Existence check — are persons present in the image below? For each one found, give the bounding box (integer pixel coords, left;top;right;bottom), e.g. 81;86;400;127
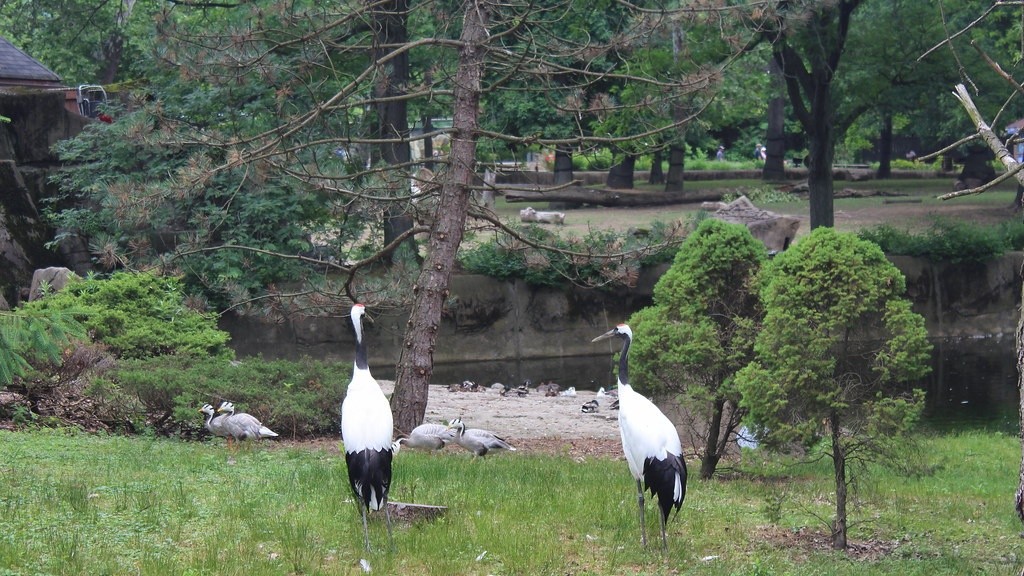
717;145;725;161
754;144;766;160
906;150;916;161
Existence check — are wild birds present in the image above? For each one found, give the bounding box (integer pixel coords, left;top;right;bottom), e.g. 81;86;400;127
341;304;394;570
581;386;613;413
593;324;687;548
563;386;577;397
447;381;511;396
393;419;516;463
514;380;562;398
198;401;279;453
